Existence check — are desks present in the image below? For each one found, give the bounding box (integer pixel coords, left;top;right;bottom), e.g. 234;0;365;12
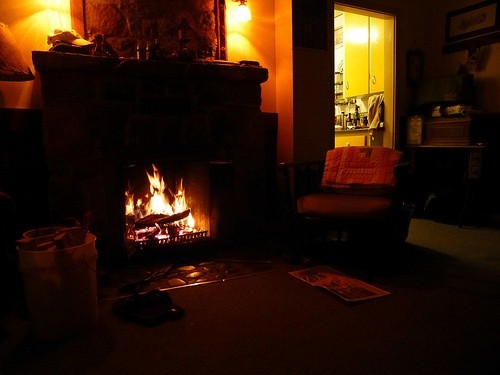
410;144;489;230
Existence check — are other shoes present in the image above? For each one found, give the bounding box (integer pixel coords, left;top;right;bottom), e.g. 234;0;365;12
140;289;183;318
113;296;162;327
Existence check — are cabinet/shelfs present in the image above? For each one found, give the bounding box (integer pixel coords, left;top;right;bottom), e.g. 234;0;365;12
342;11;370;100
334;132;369;148
369;17;385;94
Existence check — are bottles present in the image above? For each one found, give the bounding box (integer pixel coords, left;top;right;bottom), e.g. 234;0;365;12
152;31;161;60
353;105;360;126
346;113;352;130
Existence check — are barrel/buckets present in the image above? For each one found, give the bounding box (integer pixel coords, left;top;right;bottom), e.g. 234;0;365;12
0;80;33;109
18;227;98;341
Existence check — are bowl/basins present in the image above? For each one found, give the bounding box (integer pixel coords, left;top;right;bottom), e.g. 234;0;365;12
348;126;356;130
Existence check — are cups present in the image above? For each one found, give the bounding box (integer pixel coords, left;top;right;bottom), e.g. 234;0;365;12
180;49;197;62
136;39;142;59
145;40;152;61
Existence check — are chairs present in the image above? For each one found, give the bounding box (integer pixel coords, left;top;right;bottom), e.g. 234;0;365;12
279;145;417;271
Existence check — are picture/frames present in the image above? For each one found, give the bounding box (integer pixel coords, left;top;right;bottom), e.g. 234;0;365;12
444;0;500;43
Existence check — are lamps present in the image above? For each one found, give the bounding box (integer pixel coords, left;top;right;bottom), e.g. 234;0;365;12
0;24;35;108
232;0;252;22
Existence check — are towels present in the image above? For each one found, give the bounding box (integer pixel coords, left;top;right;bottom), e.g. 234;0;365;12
368;93;384;140
